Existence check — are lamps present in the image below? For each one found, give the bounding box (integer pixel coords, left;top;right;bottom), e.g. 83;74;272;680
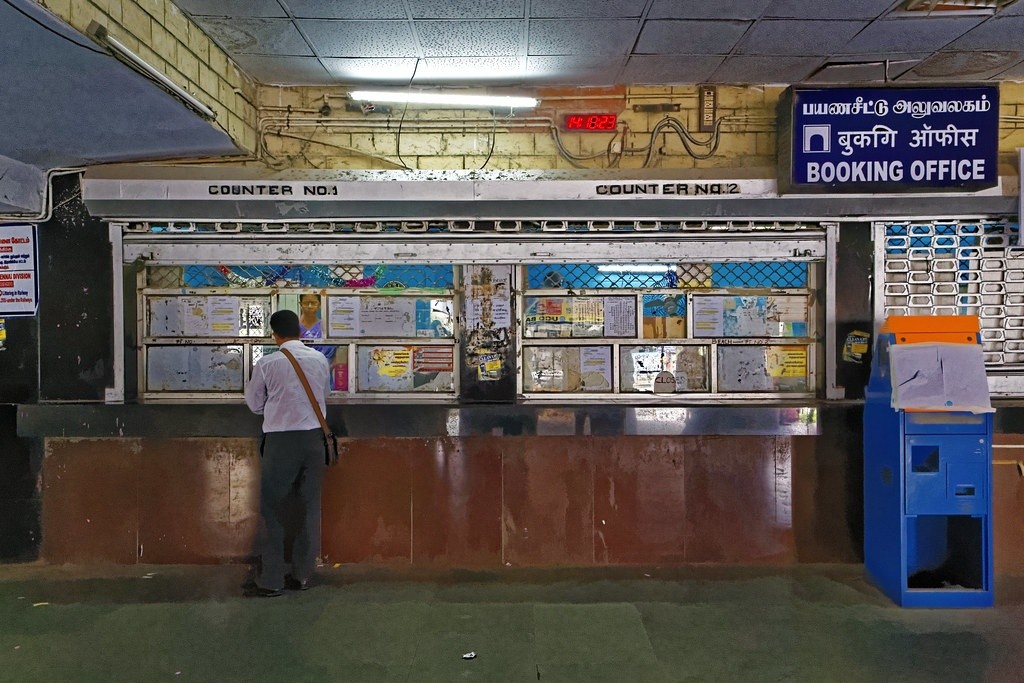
346;89;540;109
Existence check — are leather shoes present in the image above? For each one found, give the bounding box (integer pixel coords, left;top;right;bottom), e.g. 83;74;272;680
245;583;283;596
290;579;310;589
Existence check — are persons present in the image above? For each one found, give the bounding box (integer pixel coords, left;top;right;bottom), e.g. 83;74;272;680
300;294;336;390
244;309;330;596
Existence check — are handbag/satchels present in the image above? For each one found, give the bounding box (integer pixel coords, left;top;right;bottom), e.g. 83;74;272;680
324;432;338;466
